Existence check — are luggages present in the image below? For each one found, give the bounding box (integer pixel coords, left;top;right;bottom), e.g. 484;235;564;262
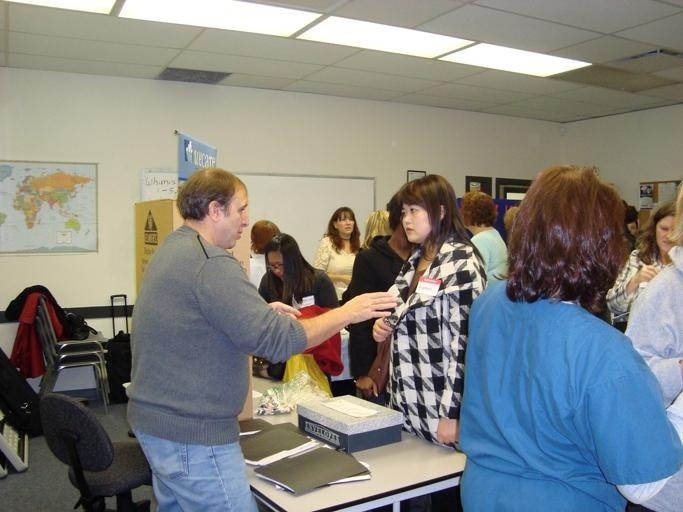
105;294;135;405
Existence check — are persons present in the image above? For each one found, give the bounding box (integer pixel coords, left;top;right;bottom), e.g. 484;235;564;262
624;178;683;444
125;168;398;512
503;206;520;235
363;208;392;250
620;199;637;250
458;163;683;512
314;207;361;300
372;175;489;452
606;202;676;333
342;189;419;406
257;233;339;385
250;219;280;290
459;191;509;287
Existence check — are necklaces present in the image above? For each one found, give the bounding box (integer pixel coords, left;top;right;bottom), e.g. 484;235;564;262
342;239;350;241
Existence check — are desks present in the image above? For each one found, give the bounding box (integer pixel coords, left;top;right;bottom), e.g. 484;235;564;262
123;377;467;512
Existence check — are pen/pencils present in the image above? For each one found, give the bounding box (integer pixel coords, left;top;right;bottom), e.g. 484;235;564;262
639;260;645;266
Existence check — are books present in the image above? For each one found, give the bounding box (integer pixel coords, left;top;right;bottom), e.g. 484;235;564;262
230;415;373;498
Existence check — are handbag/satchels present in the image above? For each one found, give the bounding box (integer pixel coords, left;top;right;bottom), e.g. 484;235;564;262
367;326;393;395
64;309;90;341
252;355;282;379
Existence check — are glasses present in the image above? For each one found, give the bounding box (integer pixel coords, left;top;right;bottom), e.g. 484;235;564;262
265;264;284;270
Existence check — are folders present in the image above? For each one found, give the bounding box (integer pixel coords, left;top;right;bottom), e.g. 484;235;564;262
241;418;372;496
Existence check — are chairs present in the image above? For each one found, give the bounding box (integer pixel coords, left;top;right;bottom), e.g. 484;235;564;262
35;298;109;416
39;394;151;512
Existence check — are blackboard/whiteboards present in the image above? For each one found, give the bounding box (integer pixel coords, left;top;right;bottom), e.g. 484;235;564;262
141;170;376;292
